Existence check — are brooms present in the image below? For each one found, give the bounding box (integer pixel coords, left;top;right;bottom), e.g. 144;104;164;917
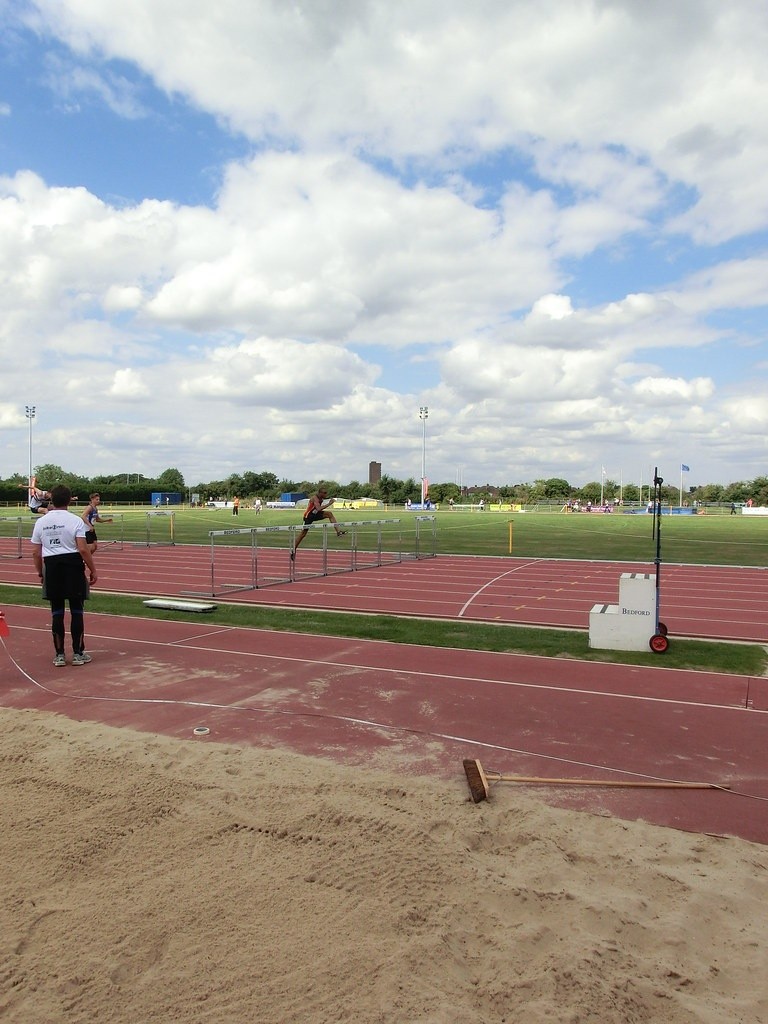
459;759;732;803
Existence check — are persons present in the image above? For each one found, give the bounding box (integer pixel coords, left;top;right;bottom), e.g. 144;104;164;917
254;495;262;515
604;499;610;513
731;503;736;515
191;499;201;507
647;500;653;513
31;485;97;666
233;496;239;516
81;492;113;564
166;496;169;504
479;498;486;510
17;483;78;515
408;497;411;506
613;497;620;506
448;497;455;510
568;498;591;512
499;499;502;511
291;488;348;561
426;497;431;507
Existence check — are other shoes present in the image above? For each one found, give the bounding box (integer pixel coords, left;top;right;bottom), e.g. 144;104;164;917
232;514;238;516
290;549;297;561
337;529;349;538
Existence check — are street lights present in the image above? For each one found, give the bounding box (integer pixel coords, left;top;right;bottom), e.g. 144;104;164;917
25;405;36;506
419;406;428;511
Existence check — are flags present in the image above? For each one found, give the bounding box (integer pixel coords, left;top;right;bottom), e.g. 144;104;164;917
682;464;689;471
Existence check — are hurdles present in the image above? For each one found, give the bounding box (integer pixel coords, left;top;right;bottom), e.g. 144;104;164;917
180;515;439;599
0;510;176;560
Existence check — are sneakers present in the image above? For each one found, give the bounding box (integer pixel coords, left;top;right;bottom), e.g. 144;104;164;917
52;653;66;667
71;651;92;666
